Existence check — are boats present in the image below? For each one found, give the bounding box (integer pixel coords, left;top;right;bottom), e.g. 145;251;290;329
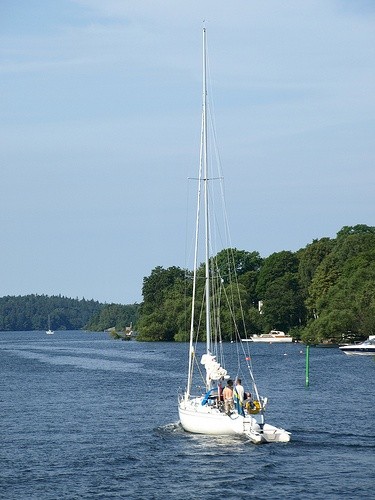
338;334;375;358
239;329;293;344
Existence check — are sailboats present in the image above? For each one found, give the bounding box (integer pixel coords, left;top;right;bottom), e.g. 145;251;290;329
176;17;296;447
45;315;54;335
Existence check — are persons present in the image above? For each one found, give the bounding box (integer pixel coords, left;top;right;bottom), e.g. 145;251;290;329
233;379;245;417
223;380;235;413
